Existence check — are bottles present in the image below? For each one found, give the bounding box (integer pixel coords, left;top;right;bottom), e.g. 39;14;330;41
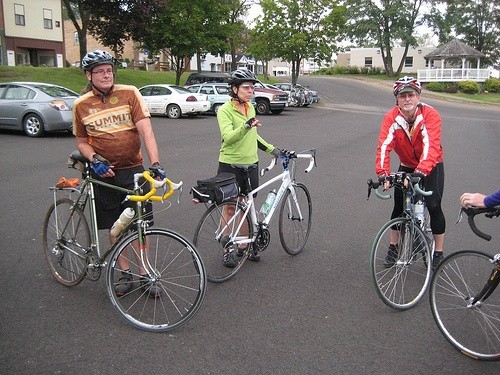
110;207;135;237
415;200;424;227
259;189;277;216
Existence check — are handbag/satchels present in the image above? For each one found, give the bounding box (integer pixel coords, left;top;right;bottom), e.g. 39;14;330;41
193;172;239;204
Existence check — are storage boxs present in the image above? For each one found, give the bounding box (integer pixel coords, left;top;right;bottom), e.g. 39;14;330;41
189;172;241;204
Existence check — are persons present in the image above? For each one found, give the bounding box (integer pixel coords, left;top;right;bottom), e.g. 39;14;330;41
72;49;165;300
459;189;500;209
376;76;445;271
216;67;280;268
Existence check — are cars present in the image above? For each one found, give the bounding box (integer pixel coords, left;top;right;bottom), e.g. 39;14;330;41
181;82;257;117
253;83;320;108
138;83;212;119
0;82;82;137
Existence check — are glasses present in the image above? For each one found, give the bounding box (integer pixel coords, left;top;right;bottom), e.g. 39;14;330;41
237;85;254;91
397;94;417;99
91;70;115;76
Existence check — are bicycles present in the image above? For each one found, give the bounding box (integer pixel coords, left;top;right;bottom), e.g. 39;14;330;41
428;205;500;361
189;148;317;284
41;150;206;332
366;171;435;312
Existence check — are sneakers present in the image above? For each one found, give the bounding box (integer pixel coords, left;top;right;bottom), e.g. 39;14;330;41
383;244;398;267
115;273;133;295
222;250;237;267
141;276;162;298
236;248;260;261
432;253;446;272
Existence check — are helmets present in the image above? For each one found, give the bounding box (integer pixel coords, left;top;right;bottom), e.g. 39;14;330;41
393;76;422;95
82;50;115;71
227;67;258;84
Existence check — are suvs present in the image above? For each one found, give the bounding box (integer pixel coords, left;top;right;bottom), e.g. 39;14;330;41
185;73;289;115
